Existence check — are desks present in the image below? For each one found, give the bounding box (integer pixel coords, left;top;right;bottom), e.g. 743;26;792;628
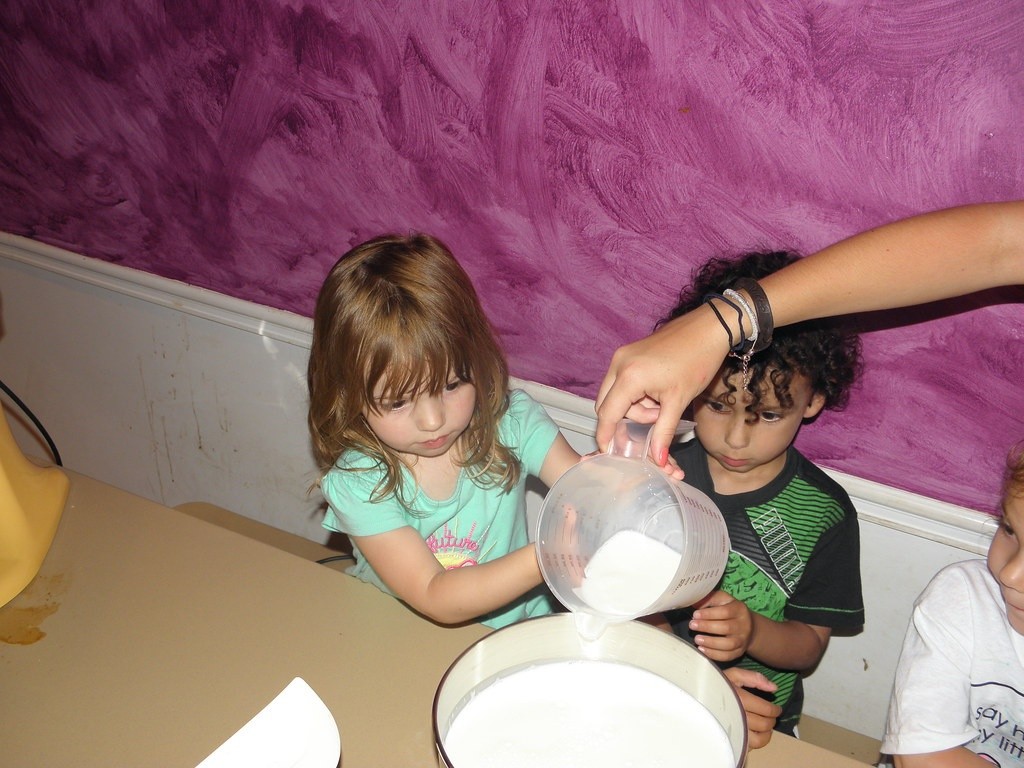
0;452;879;766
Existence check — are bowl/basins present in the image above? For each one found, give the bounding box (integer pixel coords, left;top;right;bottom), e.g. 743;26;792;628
431;611;750;768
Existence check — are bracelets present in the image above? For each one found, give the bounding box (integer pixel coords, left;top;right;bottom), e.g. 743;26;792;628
700;275;776;393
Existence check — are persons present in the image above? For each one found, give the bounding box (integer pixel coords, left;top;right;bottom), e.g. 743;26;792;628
592;199;1024;470
636;244;869;762
876;440;1024;768
304;228;683;635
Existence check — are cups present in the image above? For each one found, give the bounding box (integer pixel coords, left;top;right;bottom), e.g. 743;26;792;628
534;416;731;626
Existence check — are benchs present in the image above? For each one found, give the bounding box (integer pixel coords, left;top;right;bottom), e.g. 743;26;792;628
173;500;884;767
